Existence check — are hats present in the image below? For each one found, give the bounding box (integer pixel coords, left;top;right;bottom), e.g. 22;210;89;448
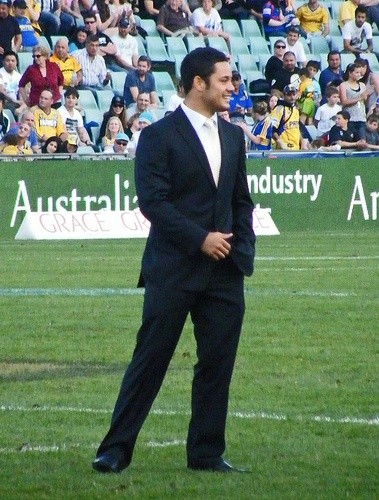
112;96;124;103
283;84;296;95
13;0;28;8
119;18;129;26
232;72;241;79
115;133;129;142
67;135;80;147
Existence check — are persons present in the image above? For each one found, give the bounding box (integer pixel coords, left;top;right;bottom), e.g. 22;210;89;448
0;0;379;156
92;46;256;473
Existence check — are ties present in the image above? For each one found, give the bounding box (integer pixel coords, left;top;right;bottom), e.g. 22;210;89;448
204;119;220;186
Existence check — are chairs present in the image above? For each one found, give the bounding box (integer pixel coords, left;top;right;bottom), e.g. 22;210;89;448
0;0;379;154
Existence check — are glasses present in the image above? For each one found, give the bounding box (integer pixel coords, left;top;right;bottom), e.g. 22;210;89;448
112;104;124;107
85;21;96;24
274;45;286;49
32;54;44;58
231;78;240;81
117;141;127;146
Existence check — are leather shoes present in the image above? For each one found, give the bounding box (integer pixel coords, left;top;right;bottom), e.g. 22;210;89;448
209;460;250;473
91;457;113;473
